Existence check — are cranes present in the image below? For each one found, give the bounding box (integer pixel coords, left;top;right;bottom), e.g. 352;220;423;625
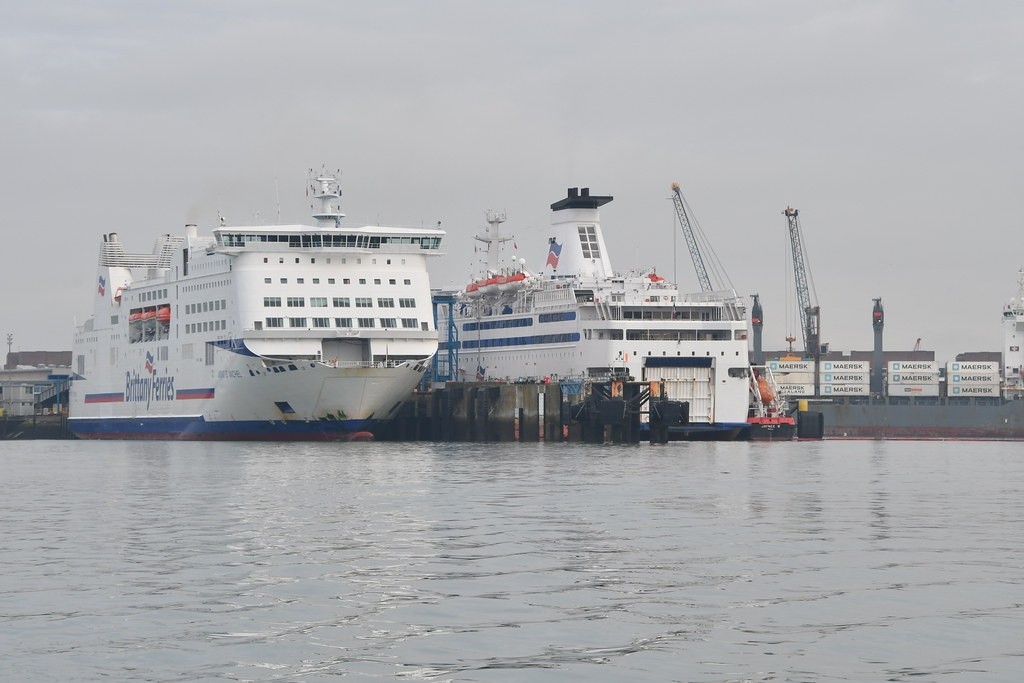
669;183;735;292
782;206;820;357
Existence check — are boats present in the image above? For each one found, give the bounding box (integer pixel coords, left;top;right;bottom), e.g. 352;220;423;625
65;163;444;440
421;186;750;438
